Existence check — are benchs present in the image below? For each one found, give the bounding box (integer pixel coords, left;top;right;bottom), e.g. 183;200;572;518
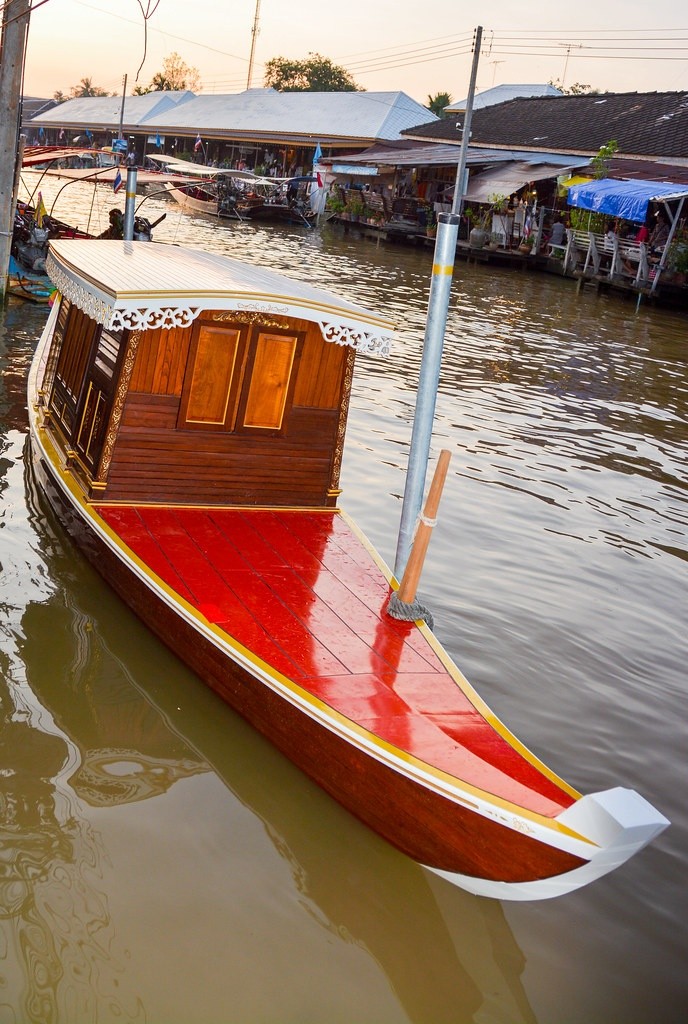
562;228;650;287
548;243;572;262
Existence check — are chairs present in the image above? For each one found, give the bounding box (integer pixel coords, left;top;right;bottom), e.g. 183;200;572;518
511;220;523;249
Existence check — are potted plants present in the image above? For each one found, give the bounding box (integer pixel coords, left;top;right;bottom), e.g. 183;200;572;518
324;182;385;226
518;234;535;253
661;223;688;284
427;224;437;238
466;192;506;251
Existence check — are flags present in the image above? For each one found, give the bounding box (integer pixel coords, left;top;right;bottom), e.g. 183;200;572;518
86;128;93;140
113;169;123;194
194;135;201;152
525;216;531;241
156;134;161;147
311;142;330;215
58;127;65;140
39;128;44;138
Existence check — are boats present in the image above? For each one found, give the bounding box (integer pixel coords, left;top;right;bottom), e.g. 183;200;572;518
4;144;216;305
145;151;320;221
23;25;676;912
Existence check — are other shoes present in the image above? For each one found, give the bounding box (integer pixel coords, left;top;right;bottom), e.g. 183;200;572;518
629;270;637;274
548;252;554;257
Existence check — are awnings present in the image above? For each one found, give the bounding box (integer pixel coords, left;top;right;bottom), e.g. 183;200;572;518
566;178;688;223
317;144;512;196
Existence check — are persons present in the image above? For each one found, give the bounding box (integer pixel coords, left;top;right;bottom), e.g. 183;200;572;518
551;216;565;252
97;208;123;239
603;221;617;254
649;213;670;252
